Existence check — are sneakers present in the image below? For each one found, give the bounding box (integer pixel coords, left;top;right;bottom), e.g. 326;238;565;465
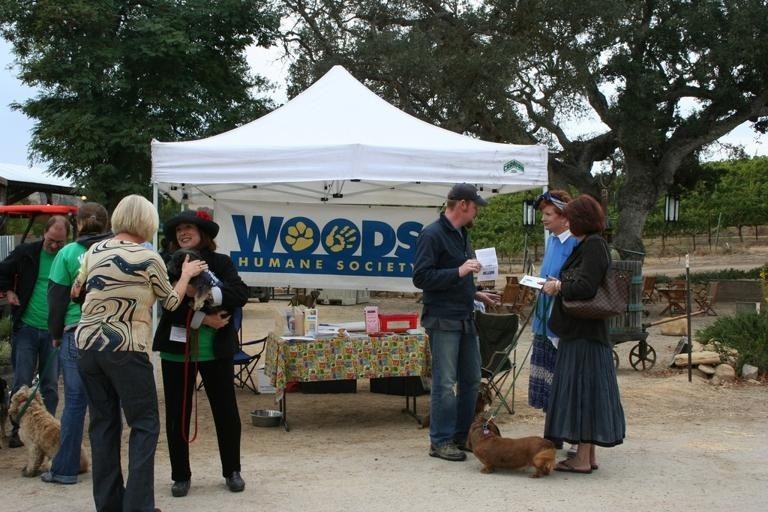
428;440;465;461
566;447;577;455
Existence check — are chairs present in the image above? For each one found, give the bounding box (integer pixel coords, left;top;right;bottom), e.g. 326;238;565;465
475;310;521;415
198;307;267;395
493;283;521;313
642;276;658;305
506;286;531;324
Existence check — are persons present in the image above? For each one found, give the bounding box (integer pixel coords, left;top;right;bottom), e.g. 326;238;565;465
152;209;250;497
536;195;626;474
528;191;579;449
40;202;113;482
1;215;71;449
69;195;209;512
412;182;501;461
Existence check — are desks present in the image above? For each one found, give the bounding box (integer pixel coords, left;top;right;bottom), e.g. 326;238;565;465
265;329;432;433
657;287;692;315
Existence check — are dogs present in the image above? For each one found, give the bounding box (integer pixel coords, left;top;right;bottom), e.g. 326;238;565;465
166;250;223;311
287;290;321;309
465;417;556;478
8;383;89;477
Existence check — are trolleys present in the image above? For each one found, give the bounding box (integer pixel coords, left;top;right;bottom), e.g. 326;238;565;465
604;248;657;372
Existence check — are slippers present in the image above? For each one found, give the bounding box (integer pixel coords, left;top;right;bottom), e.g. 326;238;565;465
566;458;598;470
553;457;592;474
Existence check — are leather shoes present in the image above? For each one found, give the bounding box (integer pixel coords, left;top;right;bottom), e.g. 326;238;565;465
41;472;76;484
171;477;191;498
226;471;246;492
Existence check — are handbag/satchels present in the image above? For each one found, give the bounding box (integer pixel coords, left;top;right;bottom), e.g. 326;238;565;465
561;240;632;317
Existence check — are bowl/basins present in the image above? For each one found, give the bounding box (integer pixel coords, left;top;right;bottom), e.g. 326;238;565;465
250;410;283;428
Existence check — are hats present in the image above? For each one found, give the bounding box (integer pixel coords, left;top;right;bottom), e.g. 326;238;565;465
163;210;219;242
447;183;488;207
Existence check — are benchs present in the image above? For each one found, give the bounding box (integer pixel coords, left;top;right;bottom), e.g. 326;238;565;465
694;281;720;316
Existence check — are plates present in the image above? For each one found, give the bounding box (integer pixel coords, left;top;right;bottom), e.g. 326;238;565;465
319;330;340;336
406;329;422;334
